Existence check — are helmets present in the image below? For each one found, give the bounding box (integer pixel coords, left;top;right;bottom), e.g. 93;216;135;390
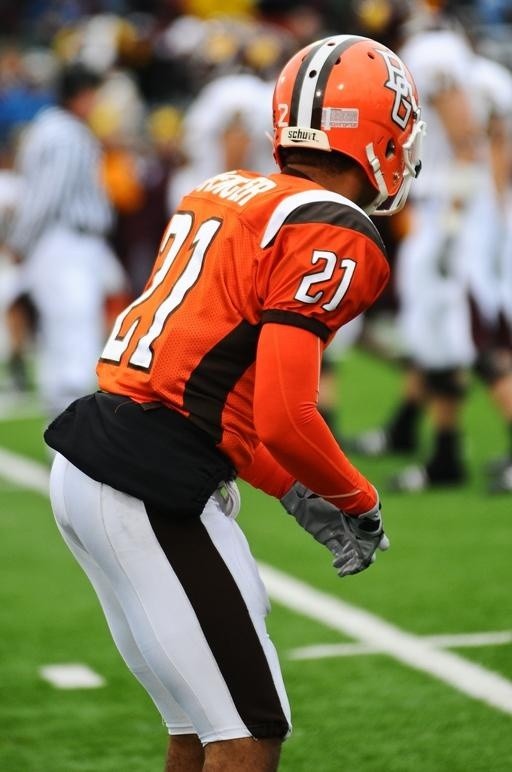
273;34;426;217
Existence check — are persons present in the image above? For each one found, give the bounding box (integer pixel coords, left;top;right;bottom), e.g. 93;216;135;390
1;0;512;494
41;31;428;772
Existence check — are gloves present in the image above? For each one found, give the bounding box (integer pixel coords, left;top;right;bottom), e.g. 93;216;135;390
280;480;388;577
